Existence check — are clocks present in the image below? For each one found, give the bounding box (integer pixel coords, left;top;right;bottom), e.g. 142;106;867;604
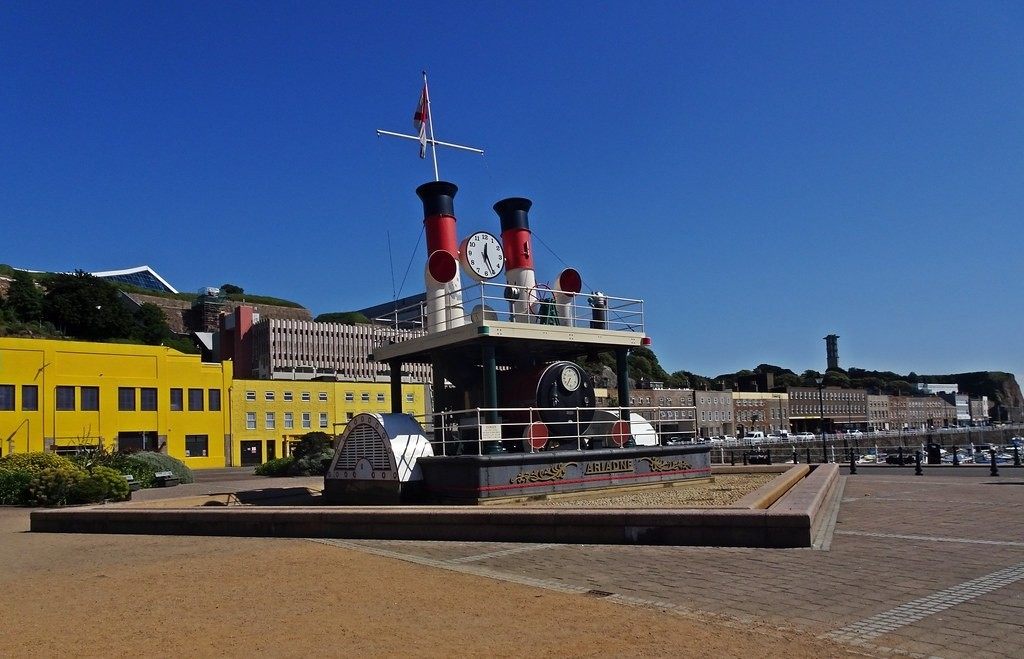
459;232;506;280
561;366;582;392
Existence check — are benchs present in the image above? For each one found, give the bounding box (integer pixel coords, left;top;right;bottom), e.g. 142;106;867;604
122;475;141;492
155;471;180;487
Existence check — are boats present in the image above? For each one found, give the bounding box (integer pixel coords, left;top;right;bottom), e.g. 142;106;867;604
854;433;1024;465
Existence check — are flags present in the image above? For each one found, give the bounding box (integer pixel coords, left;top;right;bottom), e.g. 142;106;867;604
414;86;428;159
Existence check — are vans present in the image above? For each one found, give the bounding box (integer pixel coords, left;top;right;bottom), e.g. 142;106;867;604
743;431;764;445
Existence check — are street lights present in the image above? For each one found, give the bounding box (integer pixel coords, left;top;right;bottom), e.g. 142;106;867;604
813;370;829;441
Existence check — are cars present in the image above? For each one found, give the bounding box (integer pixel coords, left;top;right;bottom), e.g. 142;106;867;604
816;431;828;440
781;432;797;443
795;432;816;442
902;423;970;432
843;429;863;440
666;434;736;446
764;433;783;444
